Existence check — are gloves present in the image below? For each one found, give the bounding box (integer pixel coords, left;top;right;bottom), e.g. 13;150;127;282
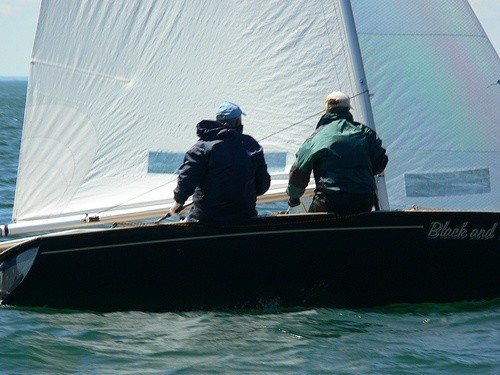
288;197;300;208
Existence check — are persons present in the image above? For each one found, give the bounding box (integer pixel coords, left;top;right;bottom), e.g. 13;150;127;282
170;101;271;225
285;91;389;214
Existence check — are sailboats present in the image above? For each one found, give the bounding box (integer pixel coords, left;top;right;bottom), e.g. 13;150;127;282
0;0;500;309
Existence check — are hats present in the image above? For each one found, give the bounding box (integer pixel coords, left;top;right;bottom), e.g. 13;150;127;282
216;102;246;121
326;91;353;110
